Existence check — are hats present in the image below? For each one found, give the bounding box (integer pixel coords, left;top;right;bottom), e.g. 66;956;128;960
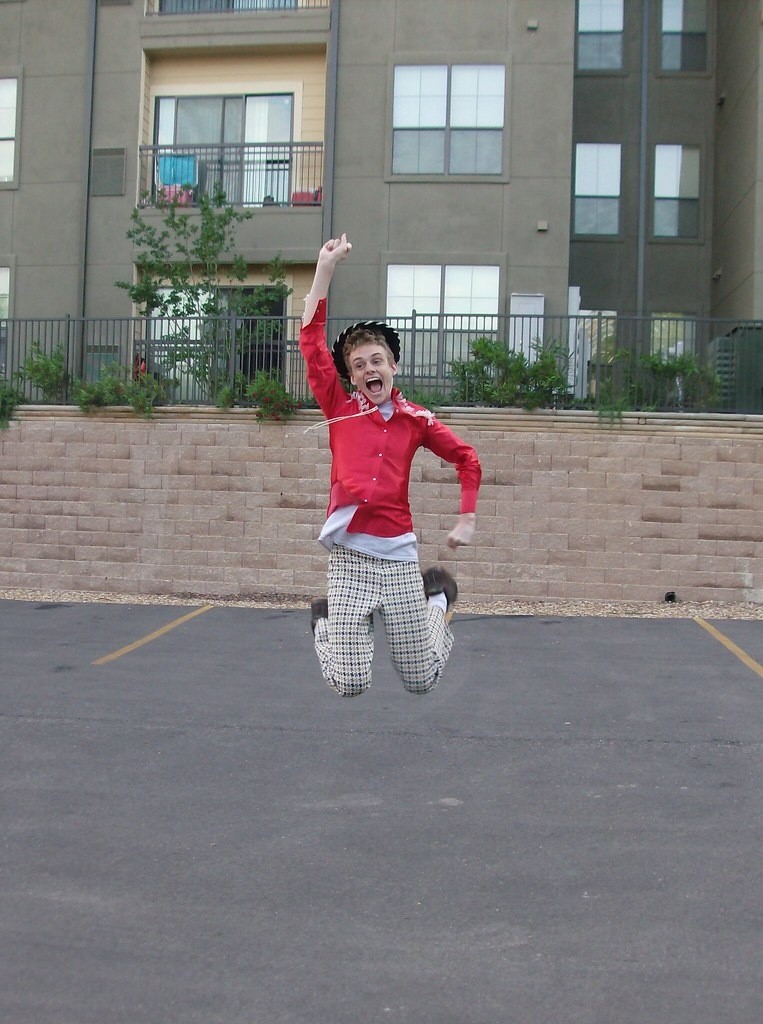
331;320;401;380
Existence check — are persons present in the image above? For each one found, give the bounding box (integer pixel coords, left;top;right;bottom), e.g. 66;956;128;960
299;233;482;697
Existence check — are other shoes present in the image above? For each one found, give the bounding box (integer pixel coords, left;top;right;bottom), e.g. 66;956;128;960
423;566;458;604
311;598;328;638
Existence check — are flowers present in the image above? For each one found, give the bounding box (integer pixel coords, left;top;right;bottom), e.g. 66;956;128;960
245;370;302;424
